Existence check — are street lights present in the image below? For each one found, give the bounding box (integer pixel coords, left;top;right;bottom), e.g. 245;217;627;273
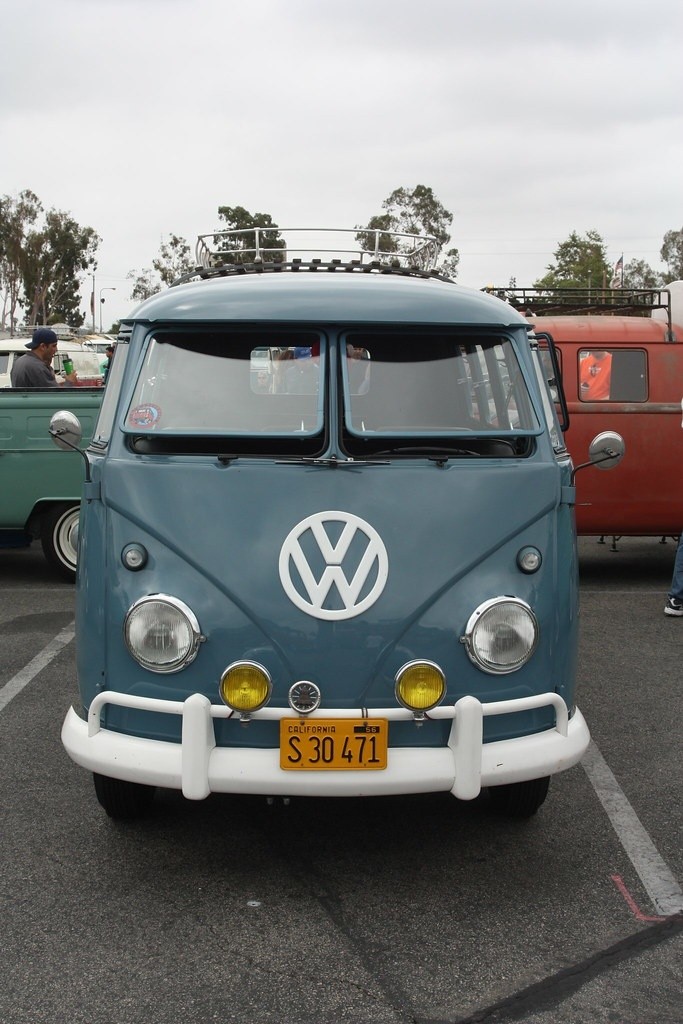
98;287;116;333
87;273;95;333
587;270;592;315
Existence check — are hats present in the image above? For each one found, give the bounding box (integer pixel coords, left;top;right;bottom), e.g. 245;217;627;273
25;328;58;349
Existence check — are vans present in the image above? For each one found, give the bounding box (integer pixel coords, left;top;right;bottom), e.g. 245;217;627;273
0;325;129;387
48;226;629;831
474;285;683;538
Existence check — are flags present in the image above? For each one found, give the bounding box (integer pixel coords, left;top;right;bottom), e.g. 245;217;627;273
615;257;623;273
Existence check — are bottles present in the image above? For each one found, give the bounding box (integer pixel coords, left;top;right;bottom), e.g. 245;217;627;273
102;362;106;370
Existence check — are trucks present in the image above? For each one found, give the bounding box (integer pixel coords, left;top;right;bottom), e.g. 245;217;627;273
1;391;106;584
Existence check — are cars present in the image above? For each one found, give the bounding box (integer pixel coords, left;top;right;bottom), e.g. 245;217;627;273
250;347;282;393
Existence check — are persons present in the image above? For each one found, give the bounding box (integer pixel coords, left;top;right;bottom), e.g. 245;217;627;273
664;529;683;615
103;346;114;369
579;350;611;401
10;327;77;387
265;343;369;395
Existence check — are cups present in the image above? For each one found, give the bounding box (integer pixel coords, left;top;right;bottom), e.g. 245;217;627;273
62;359;74;375
